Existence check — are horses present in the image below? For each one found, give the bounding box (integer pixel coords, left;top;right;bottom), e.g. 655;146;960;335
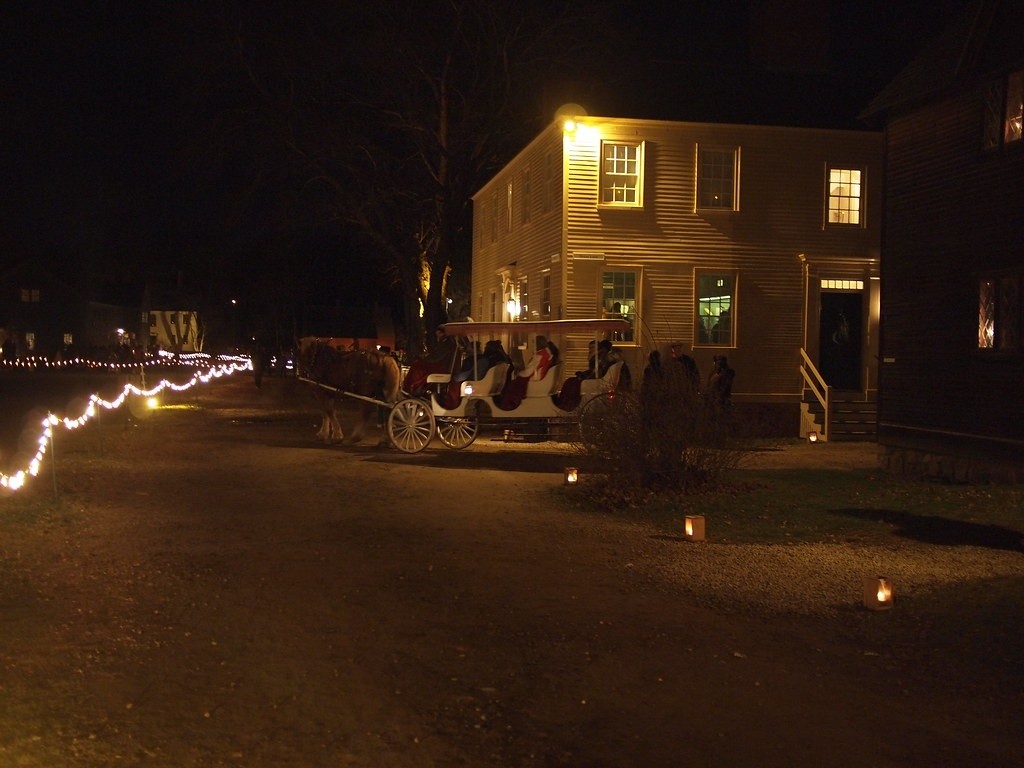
292;336;400;445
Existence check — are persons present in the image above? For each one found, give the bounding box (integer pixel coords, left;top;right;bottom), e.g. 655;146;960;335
455;336;631;402
605;301;635;341
248;348;267;387
698;304;731;344
640;353;674;405
664;344;697;422
699;351;734;444
416;324;455;391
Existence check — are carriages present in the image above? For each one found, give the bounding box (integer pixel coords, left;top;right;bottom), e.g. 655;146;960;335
247;317;646;464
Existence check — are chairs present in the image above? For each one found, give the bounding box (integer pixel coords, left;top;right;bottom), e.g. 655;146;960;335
458;359;626;397
